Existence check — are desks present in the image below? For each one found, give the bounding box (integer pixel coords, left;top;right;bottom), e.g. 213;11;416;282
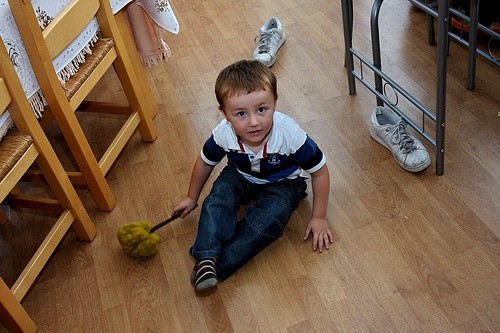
0;0;178;143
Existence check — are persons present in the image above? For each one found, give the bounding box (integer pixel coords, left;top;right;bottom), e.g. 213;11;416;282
171;59;335;297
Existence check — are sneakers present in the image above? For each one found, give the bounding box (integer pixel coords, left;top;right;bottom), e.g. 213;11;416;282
252;17;286;68
370;106;432;172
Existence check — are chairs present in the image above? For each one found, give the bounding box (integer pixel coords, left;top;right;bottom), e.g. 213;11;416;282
0;35;98;333
7;0;159;213
